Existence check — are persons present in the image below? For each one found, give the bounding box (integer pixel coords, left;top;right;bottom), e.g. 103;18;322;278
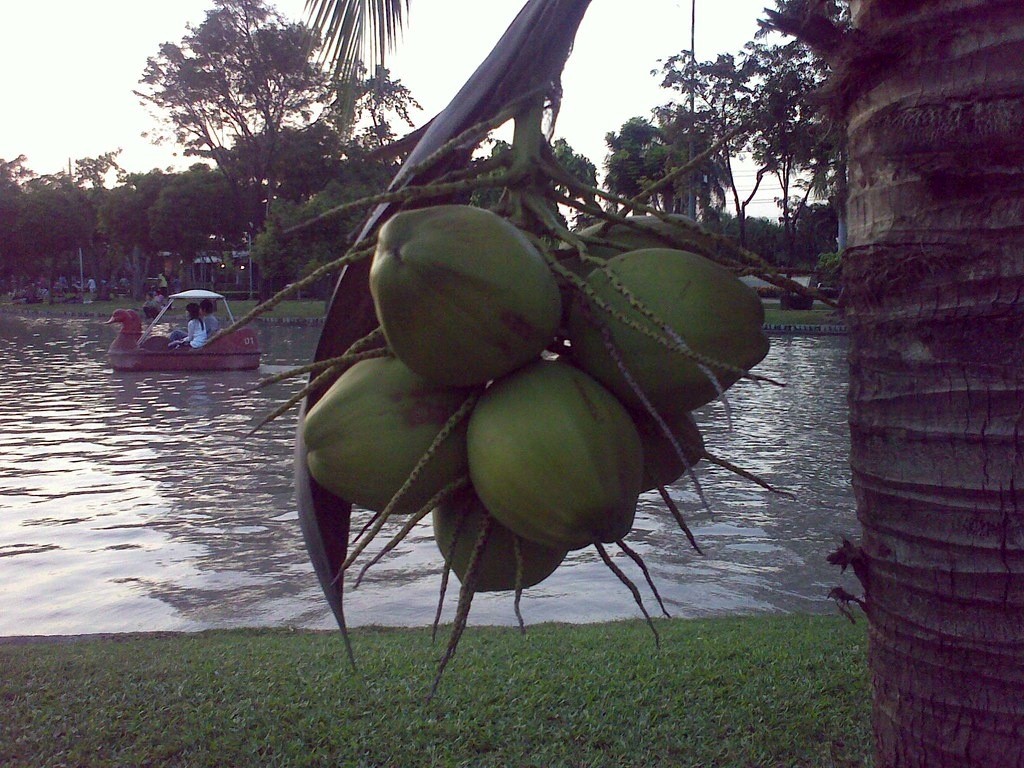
143;271;181;319
0;273;130;303
168;299;219;350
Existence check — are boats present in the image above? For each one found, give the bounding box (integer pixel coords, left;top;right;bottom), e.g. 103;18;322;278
104;289;263;371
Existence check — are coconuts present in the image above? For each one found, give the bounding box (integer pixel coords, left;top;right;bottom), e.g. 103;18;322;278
298;204;771;593
